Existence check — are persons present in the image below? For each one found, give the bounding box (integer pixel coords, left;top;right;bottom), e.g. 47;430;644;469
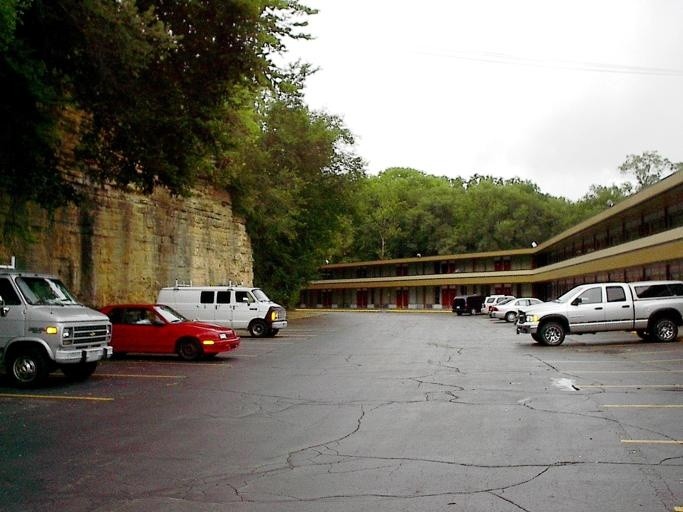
134;310;157;325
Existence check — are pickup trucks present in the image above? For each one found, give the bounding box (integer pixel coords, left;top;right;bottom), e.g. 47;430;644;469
516;280;683;347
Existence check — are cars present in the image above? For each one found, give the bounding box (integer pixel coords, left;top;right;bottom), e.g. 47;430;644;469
102;304;240;360
453;293;544;323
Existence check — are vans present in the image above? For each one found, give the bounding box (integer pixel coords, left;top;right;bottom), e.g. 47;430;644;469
156;278;287;336
0;257;114;390
